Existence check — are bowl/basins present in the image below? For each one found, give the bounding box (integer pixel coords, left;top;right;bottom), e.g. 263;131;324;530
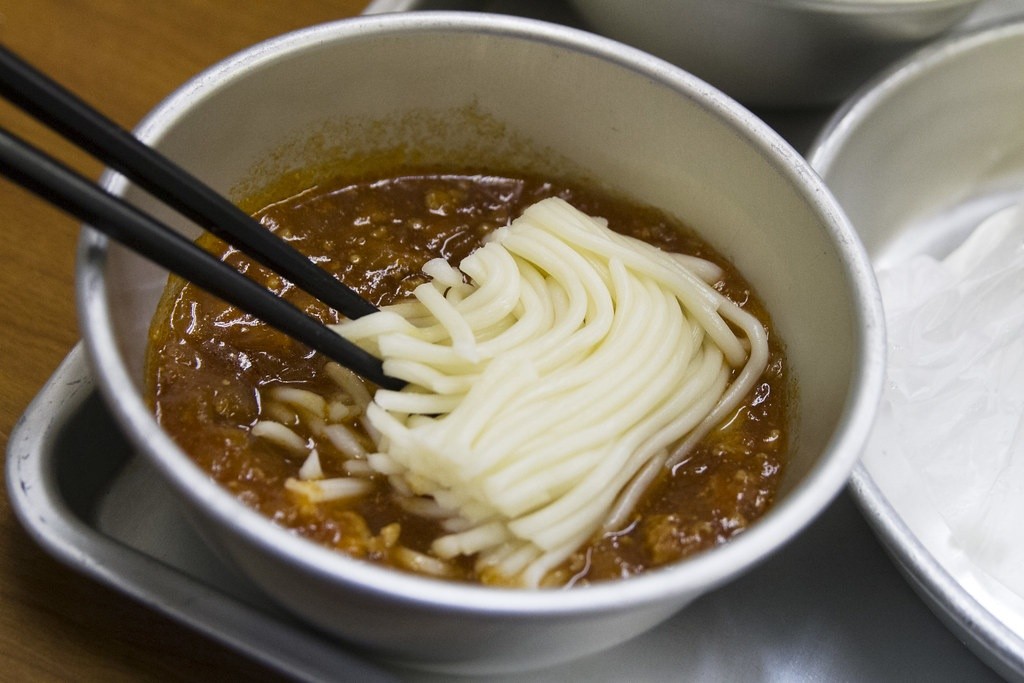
564;0;980;124
73;11;890;678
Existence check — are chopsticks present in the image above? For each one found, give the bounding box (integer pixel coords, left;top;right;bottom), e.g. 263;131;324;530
0;37;455;421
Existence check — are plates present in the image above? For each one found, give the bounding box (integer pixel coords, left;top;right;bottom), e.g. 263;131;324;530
801;14;1024;683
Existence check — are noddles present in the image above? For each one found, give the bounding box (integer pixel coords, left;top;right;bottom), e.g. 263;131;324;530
245;192;771;588
